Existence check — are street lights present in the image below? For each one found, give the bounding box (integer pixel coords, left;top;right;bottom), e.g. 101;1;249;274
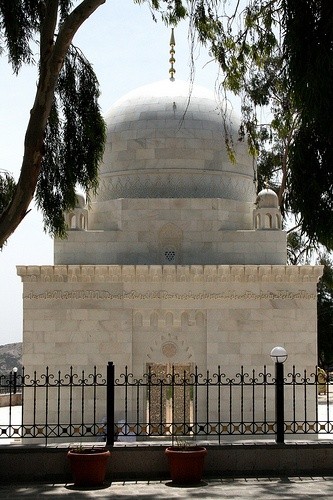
271;346;288;444
13;367;18;394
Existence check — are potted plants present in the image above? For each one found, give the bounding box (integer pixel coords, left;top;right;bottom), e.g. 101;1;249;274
165;437;207;483
67;444;110;486
